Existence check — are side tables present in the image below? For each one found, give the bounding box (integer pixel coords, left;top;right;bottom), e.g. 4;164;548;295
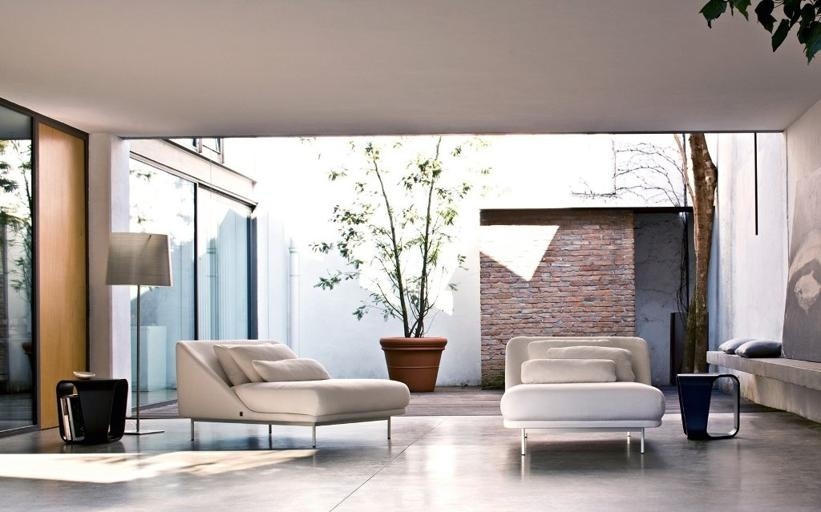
676;373;741;440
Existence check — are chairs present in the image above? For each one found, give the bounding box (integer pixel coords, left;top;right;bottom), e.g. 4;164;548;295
176;338;411;449
500;336;666;455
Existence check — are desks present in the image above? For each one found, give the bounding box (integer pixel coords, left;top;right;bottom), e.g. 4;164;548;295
56;378;129;445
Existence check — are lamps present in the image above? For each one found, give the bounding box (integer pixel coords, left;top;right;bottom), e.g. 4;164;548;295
104;232;172;435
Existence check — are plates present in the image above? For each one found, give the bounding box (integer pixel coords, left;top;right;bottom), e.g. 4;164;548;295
71;371;97;380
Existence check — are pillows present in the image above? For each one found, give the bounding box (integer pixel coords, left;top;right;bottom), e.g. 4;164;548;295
521;339;637;384
213;342;331;386
719;336;783;358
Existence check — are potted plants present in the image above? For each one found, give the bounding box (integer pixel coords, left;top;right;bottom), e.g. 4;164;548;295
299;134;494;392
